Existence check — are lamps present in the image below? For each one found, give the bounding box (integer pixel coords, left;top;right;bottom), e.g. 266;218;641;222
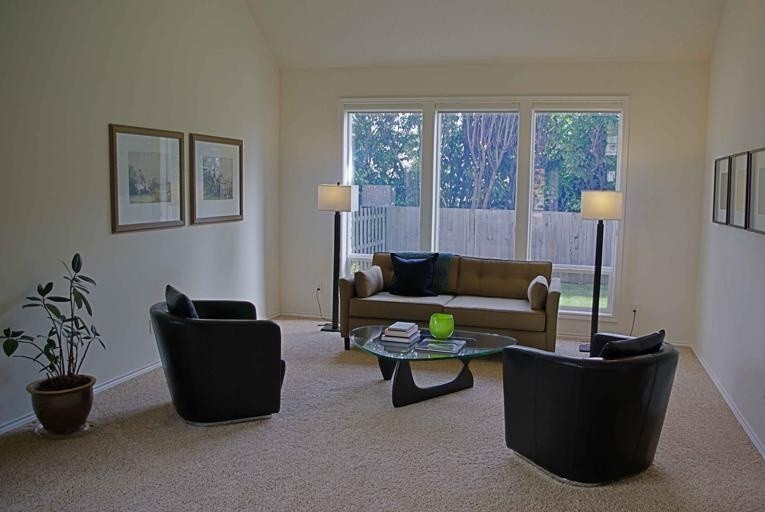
316;183;360;331
580;190;623;352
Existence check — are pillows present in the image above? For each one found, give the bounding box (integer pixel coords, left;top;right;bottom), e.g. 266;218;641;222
372;252;460;295
598;329;666;360
354;265;384;297
392;252;453;294
166;284;200;319
527;274;548;311
458;256;552;300
389;253;439;297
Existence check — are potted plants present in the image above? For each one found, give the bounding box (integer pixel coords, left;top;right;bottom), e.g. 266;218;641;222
0;253;106;435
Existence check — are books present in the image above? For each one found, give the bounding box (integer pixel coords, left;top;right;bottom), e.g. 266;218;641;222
382;342;420;354
382;321;420;343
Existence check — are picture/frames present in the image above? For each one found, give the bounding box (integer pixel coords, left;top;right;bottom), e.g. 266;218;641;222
108;124;186;233
712;147;765;234
189;133;244;224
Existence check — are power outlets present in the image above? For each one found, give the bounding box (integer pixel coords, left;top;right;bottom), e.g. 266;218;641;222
632;304;639;319
314;281;321;297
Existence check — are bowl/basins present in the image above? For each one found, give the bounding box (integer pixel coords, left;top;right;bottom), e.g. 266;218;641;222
429;312;455;339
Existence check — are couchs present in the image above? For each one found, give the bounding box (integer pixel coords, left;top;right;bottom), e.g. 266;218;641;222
339;252;561;351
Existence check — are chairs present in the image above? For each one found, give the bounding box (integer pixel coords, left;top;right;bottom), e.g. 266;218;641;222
502;333;679;487
150;301;286;426
